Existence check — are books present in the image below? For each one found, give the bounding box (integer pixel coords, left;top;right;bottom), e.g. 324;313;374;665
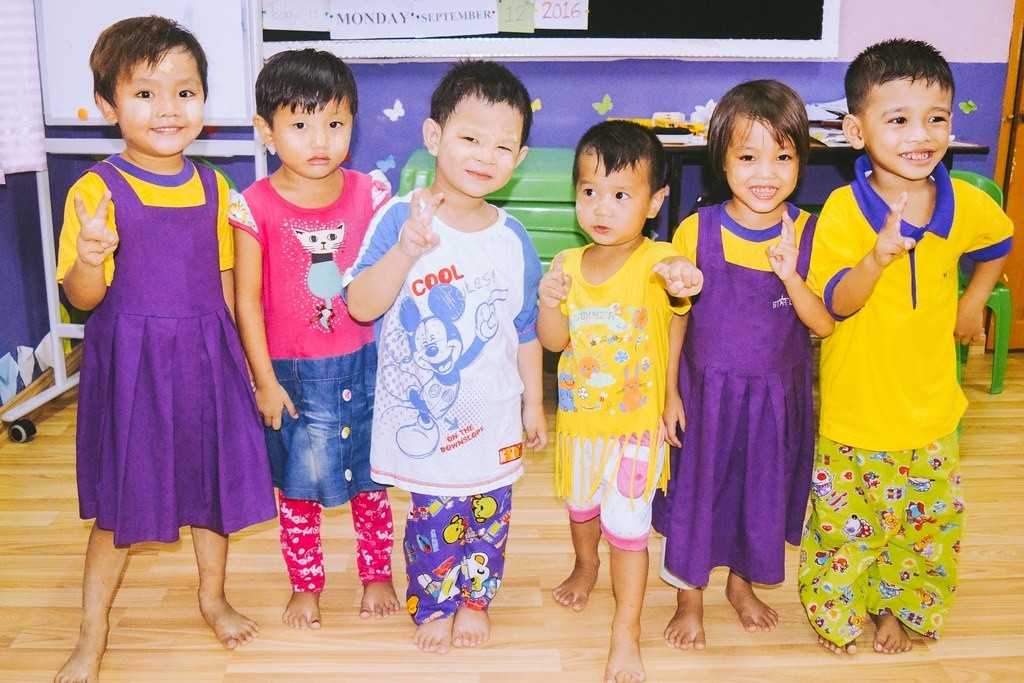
810;126;851;147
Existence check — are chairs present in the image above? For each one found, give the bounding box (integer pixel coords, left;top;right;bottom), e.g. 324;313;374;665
945;168;1012;435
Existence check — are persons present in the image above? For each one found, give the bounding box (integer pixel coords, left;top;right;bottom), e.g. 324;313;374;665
536;119;703;683
54;14;279;683
797;38;1013;653
341;60;548;654
652;79;837;648
229;48;400;629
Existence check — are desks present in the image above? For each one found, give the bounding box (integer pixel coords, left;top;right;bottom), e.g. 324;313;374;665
662;140;990;243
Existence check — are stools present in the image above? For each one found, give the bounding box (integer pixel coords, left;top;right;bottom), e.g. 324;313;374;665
398;144;594;278
